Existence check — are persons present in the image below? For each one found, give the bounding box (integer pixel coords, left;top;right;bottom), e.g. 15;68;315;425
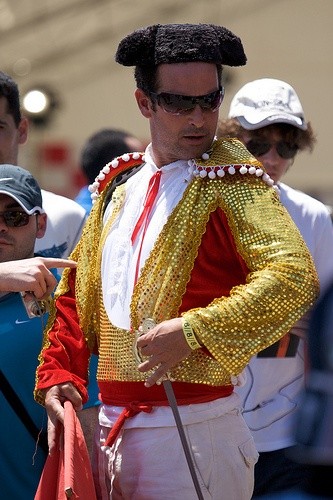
73;129;146;216
34;24;319;500
0;72;88;280
215;77;333;500
0;163;102;500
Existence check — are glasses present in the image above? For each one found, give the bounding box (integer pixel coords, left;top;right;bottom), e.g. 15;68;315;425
245;138;301;159
140;87;226;116
0;211;36;228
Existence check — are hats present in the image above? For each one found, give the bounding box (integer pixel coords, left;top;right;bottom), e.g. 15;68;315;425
0;164;45;215
115;23;247;67
228;78;310;131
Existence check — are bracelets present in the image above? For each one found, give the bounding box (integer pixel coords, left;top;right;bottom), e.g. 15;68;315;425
182;317;203;350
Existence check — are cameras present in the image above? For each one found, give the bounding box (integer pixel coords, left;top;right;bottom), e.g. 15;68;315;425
19;290;53;318
